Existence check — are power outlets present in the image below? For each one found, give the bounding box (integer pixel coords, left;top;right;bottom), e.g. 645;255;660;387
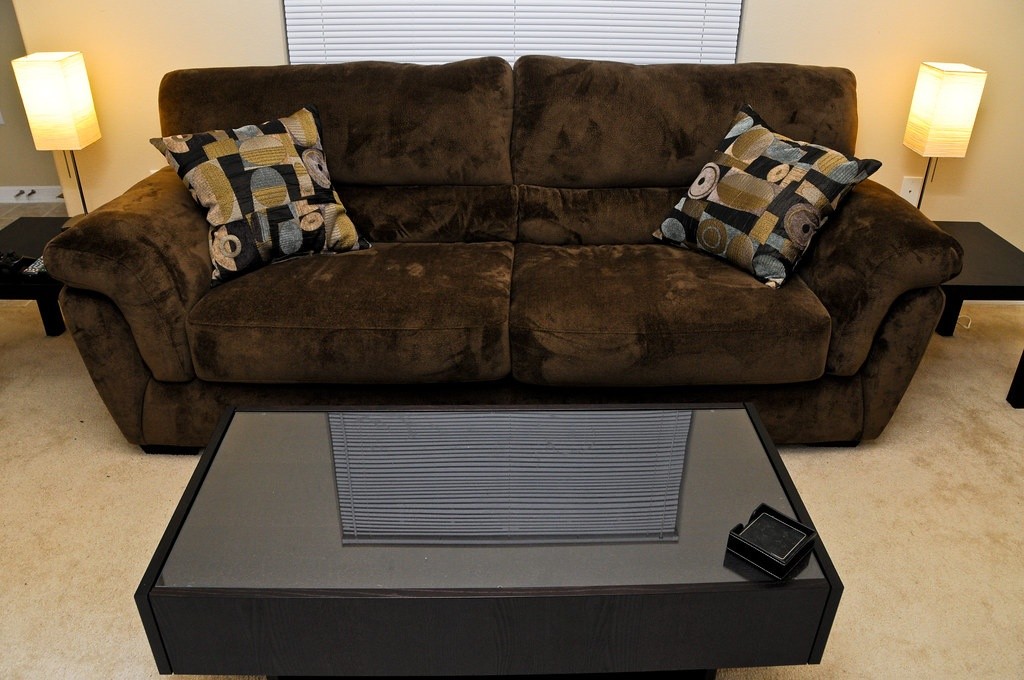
900;177;923;206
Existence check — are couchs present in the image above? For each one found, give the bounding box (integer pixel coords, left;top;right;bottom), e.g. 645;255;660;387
43;56;963;455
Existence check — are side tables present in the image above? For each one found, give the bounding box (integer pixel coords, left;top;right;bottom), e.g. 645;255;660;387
933;221;1024;409
0;217;73;337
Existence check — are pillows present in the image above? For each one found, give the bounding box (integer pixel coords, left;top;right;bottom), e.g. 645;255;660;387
662;103;882;287
150;108;372;284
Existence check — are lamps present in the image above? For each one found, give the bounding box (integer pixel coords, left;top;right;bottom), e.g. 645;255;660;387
11;51;101;230
903;61;988;211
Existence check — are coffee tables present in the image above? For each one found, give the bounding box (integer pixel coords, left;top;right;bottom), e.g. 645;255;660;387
133;400;845;680
